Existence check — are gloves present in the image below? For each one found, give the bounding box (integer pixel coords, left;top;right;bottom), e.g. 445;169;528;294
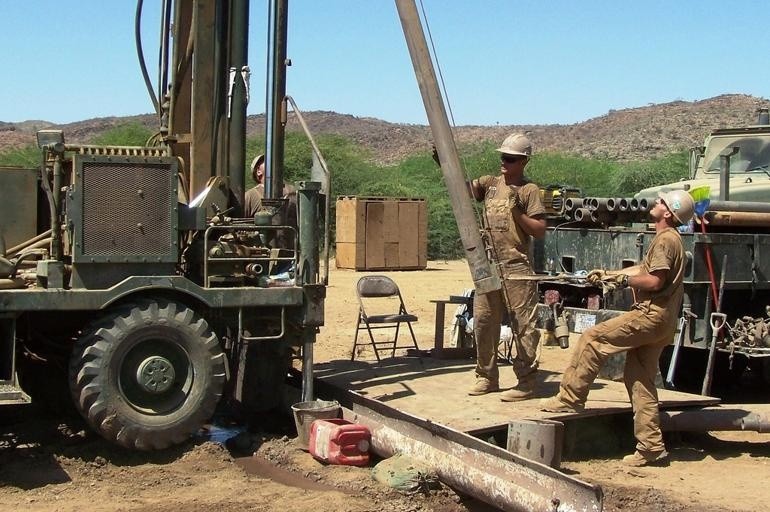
586;269;607;288
507;184;522;210
609;274;629;291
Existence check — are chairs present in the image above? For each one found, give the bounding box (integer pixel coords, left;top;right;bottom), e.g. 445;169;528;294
351;275;423;366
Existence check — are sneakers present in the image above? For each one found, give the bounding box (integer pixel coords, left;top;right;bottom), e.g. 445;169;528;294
622;447;671;466
536;396;586;413
498;380;541;402
467;374;499;397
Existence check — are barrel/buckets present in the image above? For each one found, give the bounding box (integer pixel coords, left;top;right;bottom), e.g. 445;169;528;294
292;399;341;451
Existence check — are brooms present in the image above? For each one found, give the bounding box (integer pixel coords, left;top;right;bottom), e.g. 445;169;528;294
689;185;726;348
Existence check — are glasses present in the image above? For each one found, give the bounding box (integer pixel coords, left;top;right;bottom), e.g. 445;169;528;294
500;156;522;163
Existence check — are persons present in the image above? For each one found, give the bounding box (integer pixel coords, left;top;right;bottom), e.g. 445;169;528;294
536;188;695;466
243;156;304;232
434;133;548;402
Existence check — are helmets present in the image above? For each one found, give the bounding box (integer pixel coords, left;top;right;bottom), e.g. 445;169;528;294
495;133;532;157
657;189;694;227
251;154;267;182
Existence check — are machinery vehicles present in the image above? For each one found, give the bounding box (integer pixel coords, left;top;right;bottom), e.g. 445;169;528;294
530;107;768;395
0;0;503;455
